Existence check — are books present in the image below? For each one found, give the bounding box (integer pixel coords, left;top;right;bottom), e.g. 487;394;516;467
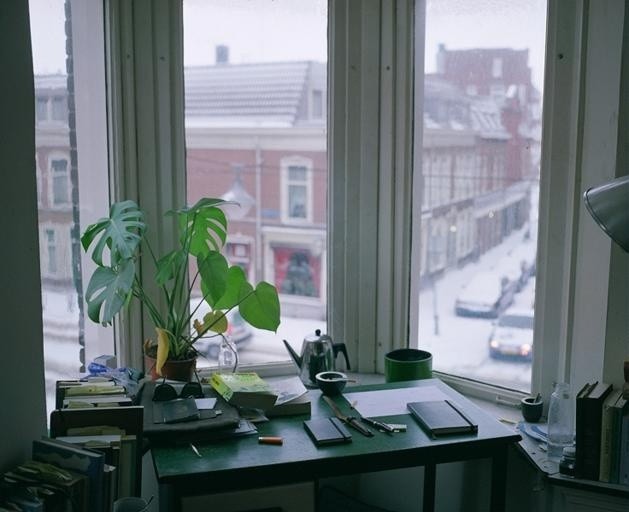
162;394;202;423
261;376;311;415
0;373;147;511
303;416;353;444
573;380;629;488
210;372;275;407
407;398;480;440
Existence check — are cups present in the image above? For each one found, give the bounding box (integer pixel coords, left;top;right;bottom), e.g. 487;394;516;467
385;348;433;382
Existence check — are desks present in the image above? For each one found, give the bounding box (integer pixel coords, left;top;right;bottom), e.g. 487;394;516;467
150;378;522;512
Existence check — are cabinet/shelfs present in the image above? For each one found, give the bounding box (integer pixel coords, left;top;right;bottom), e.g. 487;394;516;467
511;418;629;512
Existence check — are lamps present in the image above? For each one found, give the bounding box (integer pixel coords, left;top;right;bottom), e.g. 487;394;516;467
582;173;629;254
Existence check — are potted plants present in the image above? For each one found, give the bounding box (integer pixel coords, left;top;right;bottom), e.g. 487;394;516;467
79;192;280;382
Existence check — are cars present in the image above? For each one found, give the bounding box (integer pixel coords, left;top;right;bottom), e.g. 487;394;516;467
454;243;535;362
190;298;252;359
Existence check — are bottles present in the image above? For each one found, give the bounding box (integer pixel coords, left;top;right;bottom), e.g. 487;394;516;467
547;382;576;464
219;336;239;374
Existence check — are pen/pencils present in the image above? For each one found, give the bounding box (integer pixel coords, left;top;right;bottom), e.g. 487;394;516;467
360;416;393;432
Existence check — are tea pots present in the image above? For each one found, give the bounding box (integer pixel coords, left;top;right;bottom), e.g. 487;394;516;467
283;329;352;391
